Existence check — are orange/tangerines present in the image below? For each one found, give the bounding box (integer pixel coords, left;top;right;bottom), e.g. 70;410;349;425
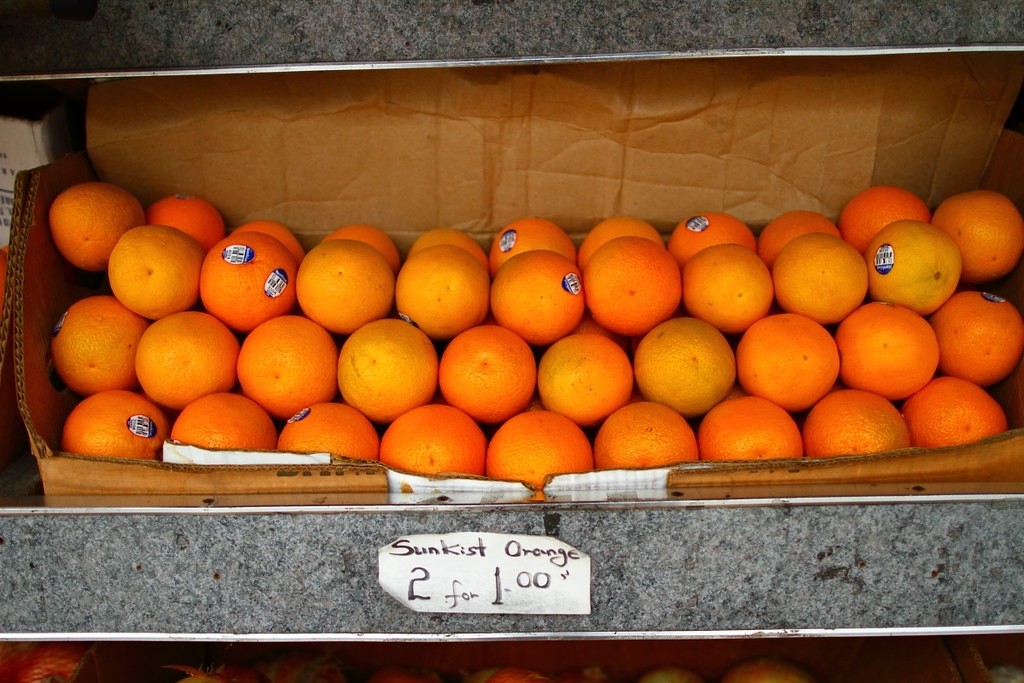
30;186;1023;501
